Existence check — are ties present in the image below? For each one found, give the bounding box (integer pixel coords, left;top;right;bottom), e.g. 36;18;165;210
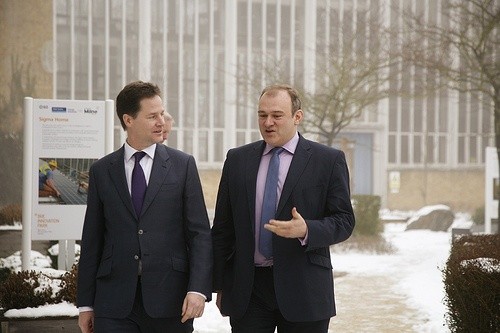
258;147;285;259
131;151;147;259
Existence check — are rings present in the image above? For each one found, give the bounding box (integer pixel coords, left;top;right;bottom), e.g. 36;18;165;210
195;313;198;316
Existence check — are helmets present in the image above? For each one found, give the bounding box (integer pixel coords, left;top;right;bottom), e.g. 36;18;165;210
49;160;57;167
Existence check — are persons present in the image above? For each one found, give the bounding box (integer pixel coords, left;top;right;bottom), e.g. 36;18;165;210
38;160;60;197
76;80;213;333
210;84;355;333
159;114;173;143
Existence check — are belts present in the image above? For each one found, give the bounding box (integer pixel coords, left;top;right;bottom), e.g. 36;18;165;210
255;266;273;273
